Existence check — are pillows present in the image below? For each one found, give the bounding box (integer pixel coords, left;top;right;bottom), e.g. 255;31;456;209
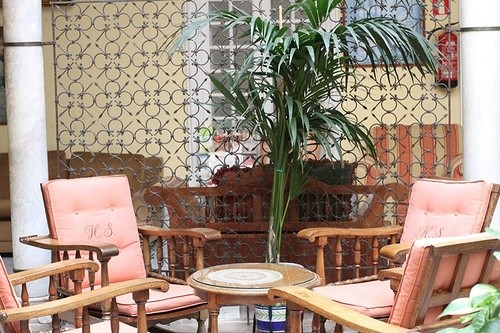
212;157;358;223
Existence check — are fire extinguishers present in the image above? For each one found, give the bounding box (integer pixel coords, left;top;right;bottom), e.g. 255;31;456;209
425;22;459;89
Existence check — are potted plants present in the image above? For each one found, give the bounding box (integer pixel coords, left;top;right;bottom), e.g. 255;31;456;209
153;0;454;333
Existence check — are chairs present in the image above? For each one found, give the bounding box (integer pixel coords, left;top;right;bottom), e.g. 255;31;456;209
1;256;170;333
21;173;222;333
297;180;499;333
267;233;500;333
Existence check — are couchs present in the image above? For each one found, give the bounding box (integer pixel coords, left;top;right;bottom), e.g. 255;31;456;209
1;149;165;255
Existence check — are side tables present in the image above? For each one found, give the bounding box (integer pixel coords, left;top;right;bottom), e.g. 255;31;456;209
187;262;321;333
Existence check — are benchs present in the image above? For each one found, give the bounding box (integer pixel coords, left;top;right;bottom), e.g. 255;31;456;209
146;183;410;283
